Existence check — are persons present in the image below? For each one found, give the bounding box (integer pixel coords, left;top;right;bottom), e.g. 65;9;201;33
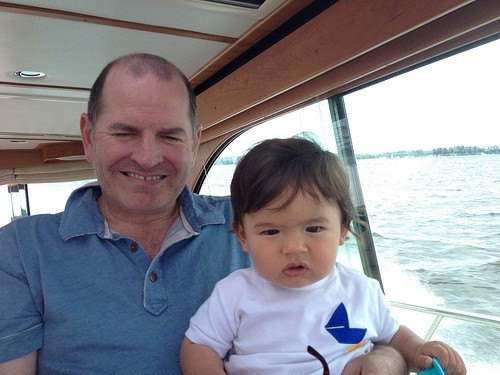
179;131;468;375
0;53;256;375
